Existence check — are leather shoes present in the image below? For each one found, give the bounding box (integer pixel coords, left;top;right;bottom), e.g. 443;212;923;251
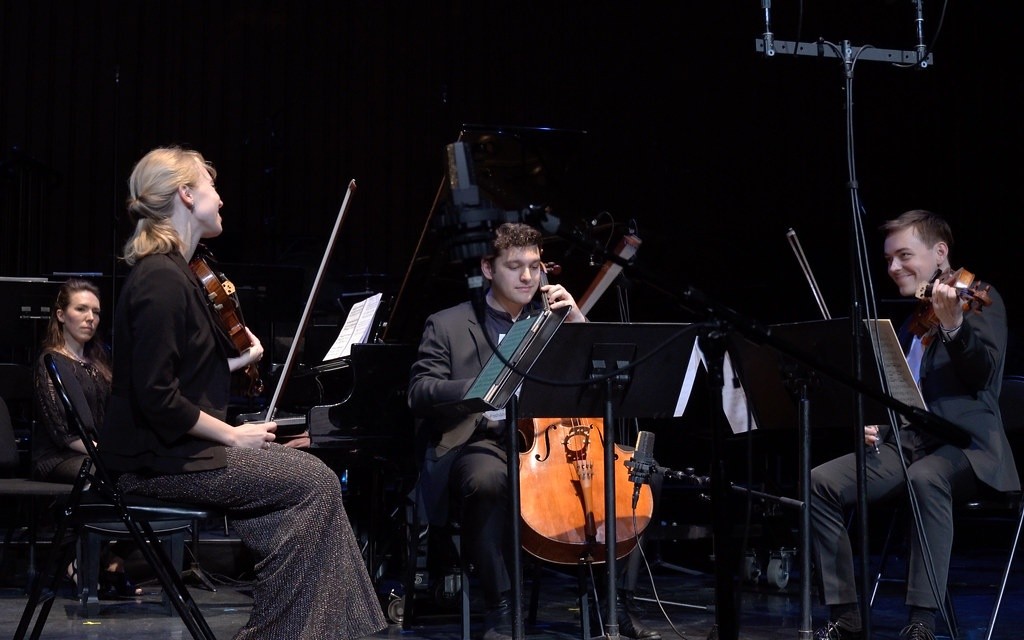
481;598;515;640
813;618;863;640
592;585;663;640
899;622;937;640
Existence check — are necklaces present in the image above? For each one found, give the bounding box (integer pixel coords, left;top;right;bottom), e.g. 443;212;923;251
65;347;98;377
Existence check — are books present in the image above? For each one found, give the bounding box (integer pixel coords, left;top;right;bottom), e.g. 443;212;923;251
864;320;928;423
322;293;384;361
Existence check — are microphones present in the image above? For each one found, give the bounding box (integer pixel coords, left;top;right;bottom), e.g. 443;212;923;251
577;217;600;237
632;431;656;508
447;143;470;231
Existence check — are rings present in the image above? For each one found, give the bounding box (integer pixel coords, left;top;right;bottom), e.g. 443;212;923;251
264;443;270;450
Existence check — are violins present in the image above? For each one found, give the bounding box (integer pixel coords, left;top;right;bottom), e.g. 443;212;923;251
188;241;265;401
909;266;997;335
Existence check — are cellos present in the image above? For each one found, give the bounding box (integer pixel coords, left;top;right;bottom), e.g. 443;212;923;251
516;260;654;638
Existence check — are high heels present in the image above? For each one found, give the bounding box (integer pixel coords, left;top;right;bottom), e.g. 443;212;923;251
63;560;99;601
103;569;141;597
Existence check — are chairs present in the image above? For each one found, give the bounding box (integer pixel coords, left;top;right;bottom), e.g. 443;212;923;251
869;376;1024;640
394;381;551;640
0;393;89;598
12;353;217;640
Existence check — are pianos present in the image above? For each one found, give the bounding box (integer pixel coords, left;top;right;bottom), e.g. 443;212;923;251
231;292;498;622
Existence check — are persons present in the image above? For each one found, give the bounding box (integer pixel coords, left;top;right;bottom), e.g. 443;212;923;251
0;396;18;479
101;146;389;640
407;222;661;640
811;210;1021;640
32;279;152;598
